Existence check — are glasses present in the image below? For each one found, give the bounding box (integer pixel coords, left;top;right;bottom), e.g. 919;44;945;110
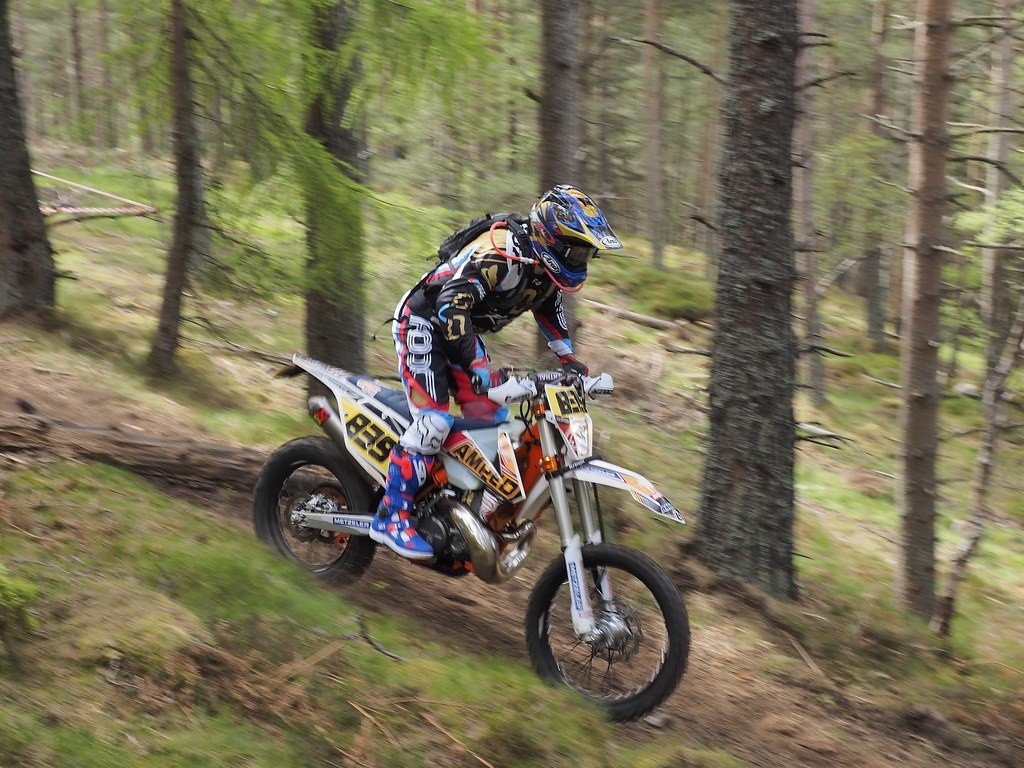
559;243;597;270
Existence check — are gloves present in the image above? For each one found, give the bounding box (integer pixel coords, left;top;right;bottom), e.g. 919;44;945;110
561;354;588;379
470;358;510;395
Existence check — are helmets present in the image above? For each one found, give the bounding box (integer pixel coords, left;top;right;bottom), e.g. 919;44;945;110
528;184;623;289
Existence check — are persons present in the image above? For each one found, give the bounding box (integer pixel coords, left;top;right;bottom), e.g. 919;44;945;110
368;182;623;561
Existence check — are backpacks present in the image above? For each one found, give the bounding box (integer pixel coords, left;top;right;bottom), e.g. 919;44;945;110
425;212;533;276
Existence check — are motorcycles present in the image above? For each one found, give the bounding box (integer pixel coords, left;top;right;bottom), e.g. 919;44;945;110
246;350;691;723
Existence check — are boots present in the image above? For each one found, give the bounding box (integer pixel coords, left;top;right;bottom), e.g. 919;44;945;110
370;444;434;559
